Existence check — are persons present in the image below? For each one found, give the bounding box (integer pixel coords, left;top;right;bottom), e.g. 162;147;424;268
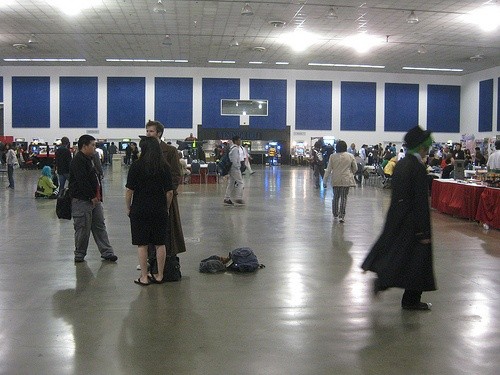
326;142;406;184
15;148;46;170
55;137;73;195
69;135;118;263
125;121;186;286
428;140;500;179
5;143;20;191
323;141;358;223
37;166;57;197
214;136;255;205
361;126;438;311
95;141;118;166
312;139;327;189
269;145;276;165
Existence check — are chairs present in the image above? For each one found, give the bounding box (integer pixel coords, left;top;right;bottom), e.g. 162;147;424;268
190;163;202;185
204;162;219;185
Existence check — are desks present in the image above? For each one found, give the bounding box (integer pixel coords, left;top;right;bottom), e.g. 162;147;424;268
180;164;219;185
474;186;500;231
430;178;487;223
36;154;57;170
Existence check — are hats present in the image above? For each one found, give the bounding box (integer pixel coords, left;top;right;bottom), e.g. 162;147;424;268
404;126;432;148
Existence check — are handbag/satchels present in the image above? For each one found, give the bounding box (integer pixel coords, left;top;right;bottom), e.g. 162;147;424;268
151;256;181;280
228;247;259;273
56;188;72;220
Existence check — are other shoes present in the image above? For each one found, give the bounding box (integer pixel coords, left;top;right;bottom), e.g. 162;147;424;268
102;255;118;262
235;200;245;205
339;217;344;222
75;257;84;262
251;172;256;175
402;302;432;310
334;215;339;219
224;199;233;205
241;172;246;175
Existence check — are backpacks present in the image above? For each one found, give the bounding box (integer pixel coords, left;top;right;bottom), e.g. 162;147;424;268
218;153;232;175
313;148;325;165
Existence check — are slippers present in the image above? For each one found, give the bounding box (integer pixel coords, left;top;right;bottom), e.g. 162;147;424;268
134;277;149;285
147;273;162;283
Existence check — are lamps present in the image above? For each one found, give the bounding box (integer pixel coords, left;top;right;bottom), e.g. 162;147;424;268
239;1;255;17
417;44;429;54
28;33;41;45
229;36;239;47
161;34;173;46
406;11;418;24
152;0;167;15
324;6;338;19
